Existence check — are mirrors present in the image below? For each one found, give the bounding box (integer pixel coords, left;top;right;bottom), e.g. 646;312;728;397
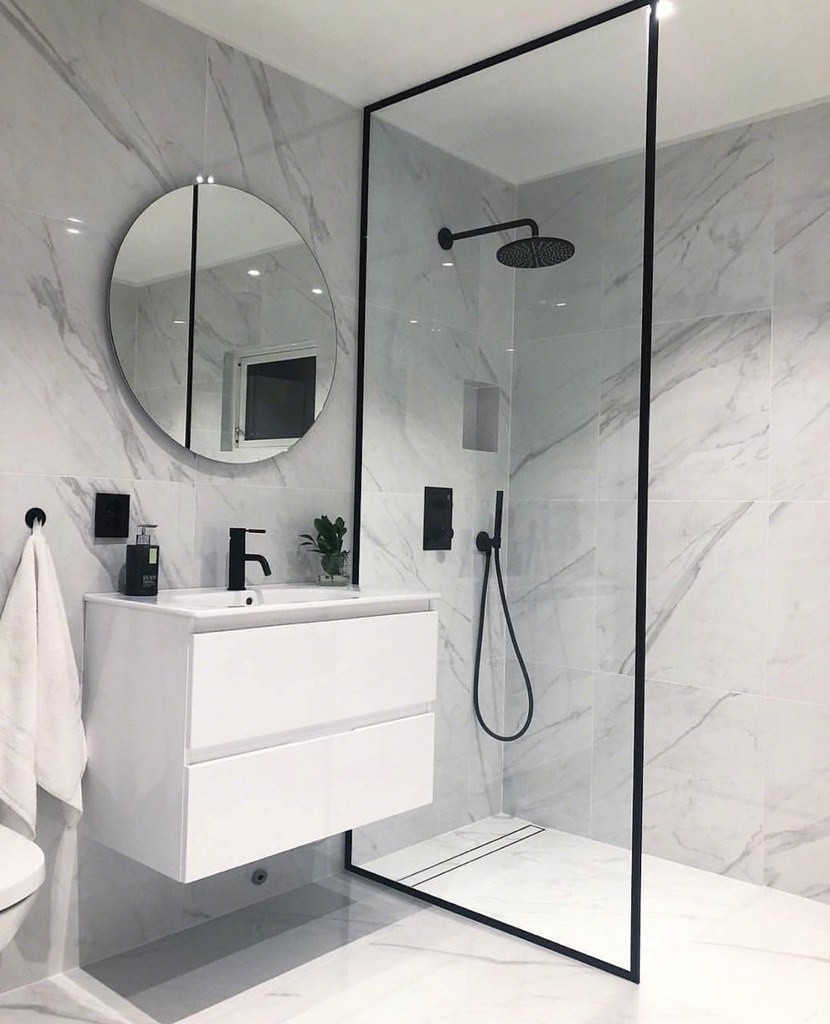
108;179;340;465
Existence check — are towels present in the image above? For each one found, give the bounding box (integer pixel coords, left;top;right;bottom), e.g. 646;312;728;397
0;530;91;849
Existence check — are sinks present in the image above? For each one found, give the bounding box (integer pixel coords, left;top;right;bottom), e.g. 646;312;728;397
174;586;368;613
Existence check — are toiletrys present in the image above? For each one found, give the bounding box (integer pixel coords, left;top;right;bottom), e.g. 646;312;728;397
124;523;162;597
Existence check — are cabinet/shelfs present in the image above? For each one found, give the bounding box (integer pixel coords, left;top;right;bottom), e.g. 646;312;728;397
79;607;442;883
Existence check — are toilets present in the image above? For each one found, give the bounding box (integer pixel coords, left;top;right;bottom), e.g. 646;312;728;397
0;820;48;956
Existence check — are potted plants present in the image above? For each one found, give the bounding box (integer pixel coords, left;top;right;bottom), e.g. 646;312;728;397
298;514;351;586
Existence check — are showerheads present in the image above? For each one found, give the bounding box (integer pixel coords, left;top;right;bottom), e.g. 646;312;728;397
495;489;506;547
495;235;576;270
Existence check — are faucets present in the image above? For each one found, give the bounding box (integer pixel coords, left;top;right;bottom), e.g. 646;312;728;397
226;526;273;591
